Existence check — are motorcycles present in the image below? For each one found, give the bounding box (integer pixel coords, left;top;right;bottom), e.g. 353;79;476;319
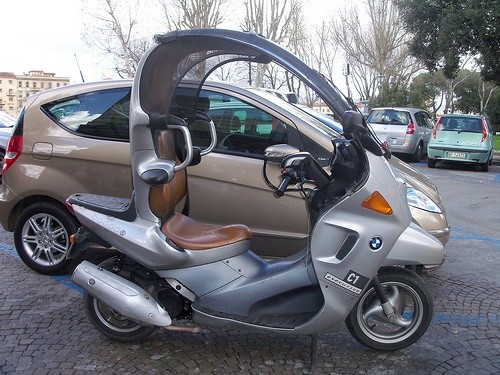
63;27;448;354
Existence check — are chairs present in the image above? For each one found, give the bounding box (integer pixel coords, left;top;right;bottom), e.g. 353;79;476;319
187;96;223;149
470;122;480;132
443;119;459;130
228;116;252;153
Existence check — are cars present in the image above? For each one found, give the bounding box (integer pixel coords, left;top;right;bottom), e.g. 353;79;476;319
427;113;494;172
209;101;289;141
367;107;432;160
0;80;452;277
0;110;17;173
